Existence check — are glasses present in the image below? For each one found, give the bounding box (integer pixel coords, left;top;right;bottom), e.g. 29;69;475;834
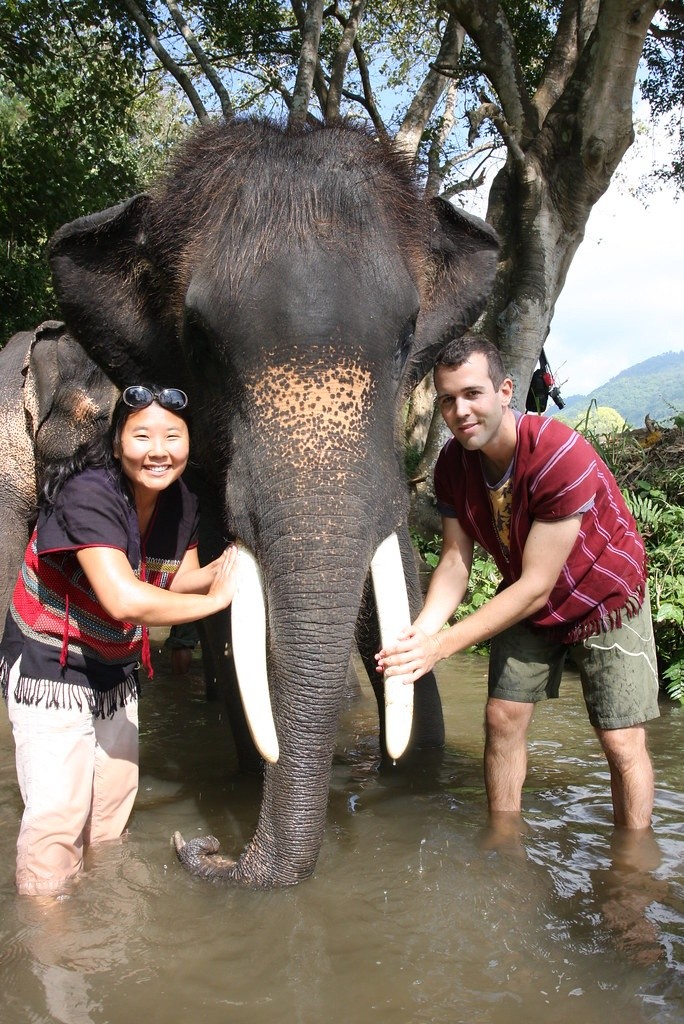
118;386;188;411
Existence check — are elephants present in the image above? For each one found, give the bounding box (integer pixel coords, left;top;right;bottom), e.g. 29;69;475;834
51;120;500;890
0;322;124;655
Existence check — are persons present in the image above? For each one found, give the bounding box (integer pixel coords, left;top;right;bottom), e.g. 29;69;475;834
374;337;661;829
0;382;241;895
